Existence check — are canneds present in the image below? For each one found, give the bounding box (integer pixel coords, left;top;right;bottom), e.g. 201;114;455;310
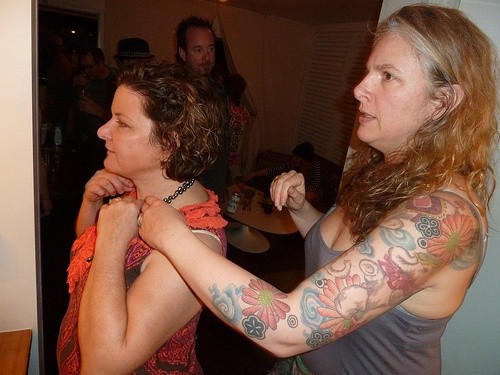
226;192;240;213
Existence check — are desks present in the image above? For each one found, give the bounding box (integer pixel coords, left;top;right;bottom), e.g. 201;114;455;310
220;186;301;253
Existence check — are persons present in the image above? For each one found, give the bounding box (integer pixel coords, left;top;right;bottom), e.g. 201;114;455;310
242;142;321;196
40;17;246;294
56;59;228;375
137;3;500;374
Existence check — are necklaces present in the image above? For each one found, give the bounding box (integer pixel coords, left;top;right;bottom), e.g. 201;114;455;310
163;178;195;204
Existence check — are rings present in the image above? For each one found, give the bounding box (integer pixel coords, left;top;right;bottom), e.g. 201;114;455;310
137;213;142;227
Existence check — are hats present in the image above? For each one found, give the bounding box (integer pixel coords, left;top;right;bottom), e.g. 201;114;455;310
114;37;156;60
293;142;314;162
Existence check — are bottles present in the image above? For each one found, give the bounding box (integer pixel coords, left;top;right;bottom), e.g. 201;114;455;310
240;189;254;216
263;190;273;214
54;124;63;146
